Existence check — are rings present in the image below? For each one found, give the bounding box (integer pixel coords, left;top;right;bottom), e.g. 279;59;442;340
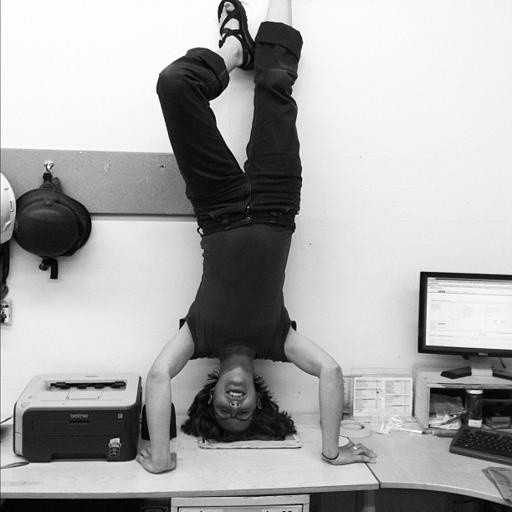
352;445;357;451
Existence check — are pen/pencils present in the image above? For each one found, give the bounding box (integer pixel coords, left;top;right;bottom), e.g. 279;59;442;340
389;426;426;434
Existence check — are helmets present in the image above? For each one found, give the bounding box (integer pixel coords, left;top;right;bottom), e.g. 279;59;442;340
13;182;91;279
0;172;16;245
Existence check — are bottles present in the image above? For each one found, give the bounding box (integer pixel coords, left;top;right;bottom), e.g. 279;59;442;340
464;390;484;428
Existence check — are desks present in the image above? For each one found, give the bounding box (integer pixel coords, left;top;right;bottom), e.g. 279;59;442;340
0;415;512;506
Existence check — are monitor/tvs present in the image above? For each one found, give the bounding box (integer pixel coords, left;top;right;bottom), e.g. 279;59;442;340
418;271;512;381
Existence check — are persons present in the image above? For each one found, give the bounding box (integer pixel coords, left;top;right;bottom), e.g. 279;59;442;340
136;0;376;474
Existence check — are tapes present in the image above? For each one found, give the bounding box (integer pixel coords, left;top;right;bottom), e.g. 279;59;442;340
339;419;371;438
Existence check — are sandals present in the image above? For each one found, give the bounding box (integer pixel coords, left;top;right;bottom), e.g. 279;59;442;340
218;0;255;71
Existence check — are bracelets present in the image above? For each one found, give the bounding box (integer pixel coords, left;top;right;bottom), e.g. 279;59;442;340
321;452;339;460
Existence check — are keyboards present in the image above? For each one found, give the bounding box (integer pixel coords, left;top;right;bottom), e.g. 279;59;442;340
449;425;512;465
